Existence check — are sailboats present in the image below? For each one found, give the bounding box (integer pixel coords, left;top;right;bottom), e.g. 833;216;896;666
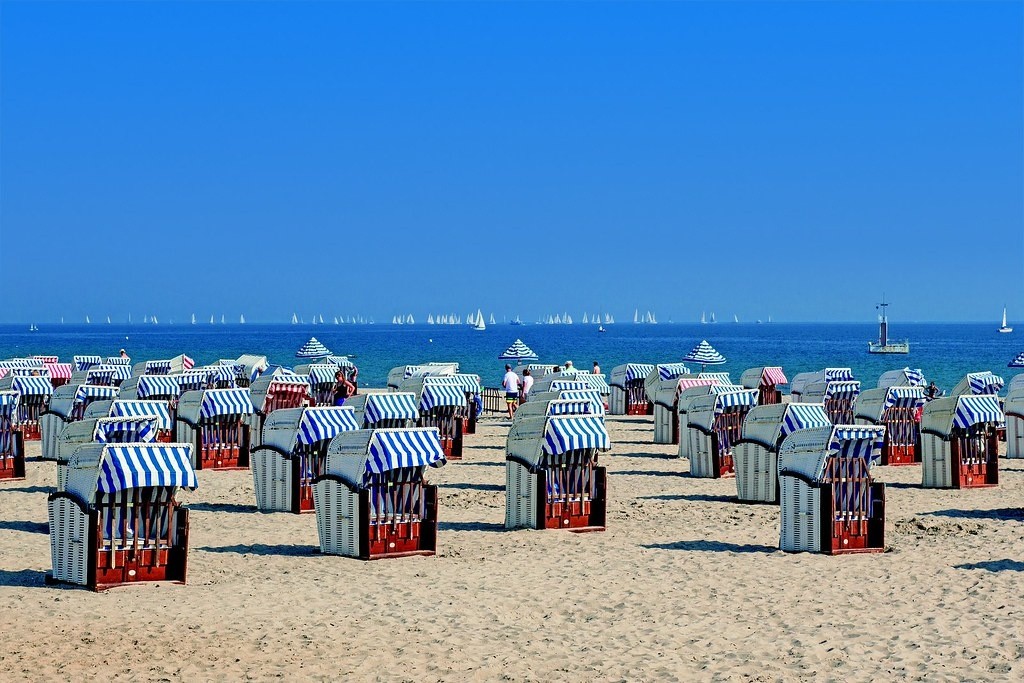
470;308;486;331
24;309;742;333
996;305;1014;333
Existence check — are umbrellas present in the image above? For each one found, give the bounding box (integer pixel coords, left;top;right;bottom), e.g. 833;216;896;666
683;341;726;373
498;338;538;366
295;337;334;364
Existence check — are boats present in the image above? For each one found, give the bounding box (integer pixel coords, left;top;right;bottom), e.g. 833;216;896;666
868;292;909;353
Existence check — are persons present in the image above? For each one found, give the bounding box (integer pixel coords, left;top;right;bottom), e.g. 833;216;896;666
592;361;600;374
118;348;129;357
332;371;355;406
926;382;939;398
502;364;534;420
564;361;578;372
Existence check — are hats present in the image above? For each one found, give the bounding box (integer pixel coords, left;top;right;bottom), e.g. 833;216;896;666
120;349;125;352
